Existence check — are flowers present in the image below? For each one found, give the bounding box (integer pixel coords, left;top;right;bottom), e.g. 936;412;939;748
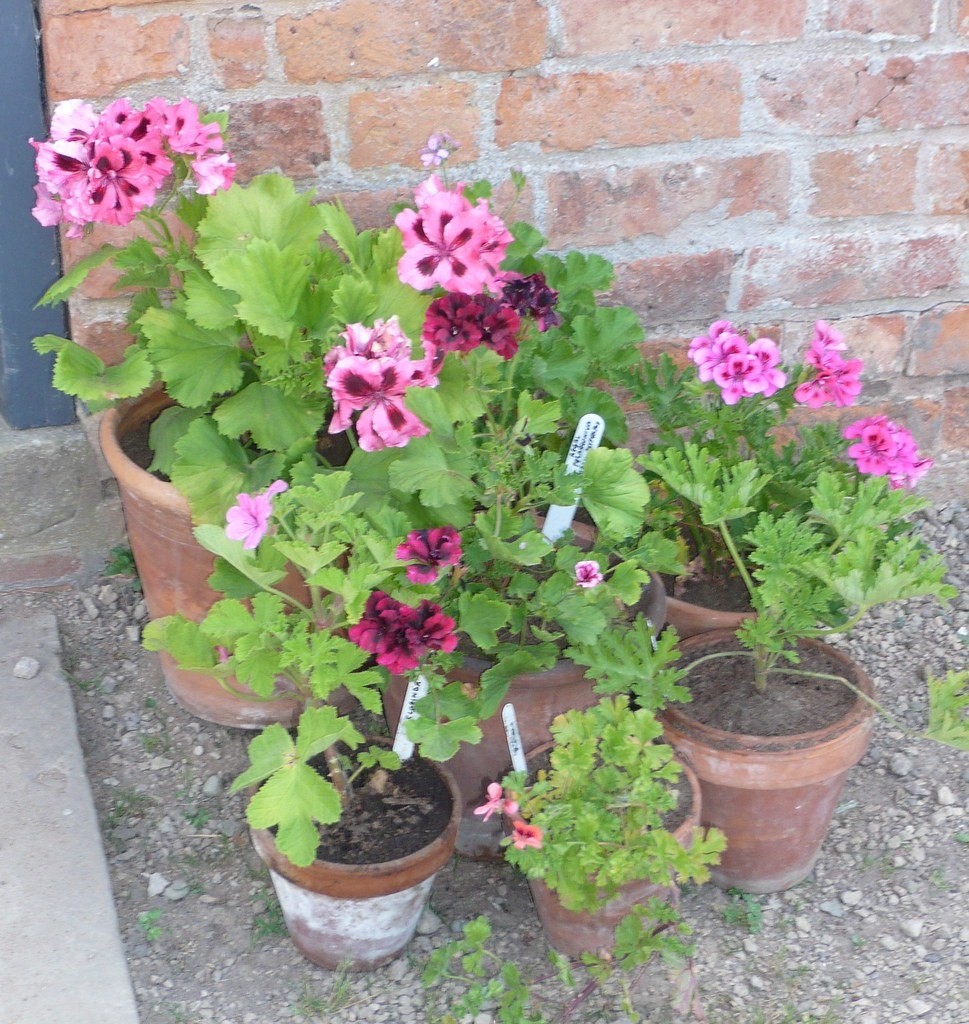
30;96;969;1024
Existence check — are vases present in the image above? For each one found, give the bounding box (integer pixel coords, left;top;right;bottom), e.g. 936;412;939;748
508;742;701;959
246;735;464;969
664;502;784;642
648;630;879;891
385;513;674;858
97;380;362;729
